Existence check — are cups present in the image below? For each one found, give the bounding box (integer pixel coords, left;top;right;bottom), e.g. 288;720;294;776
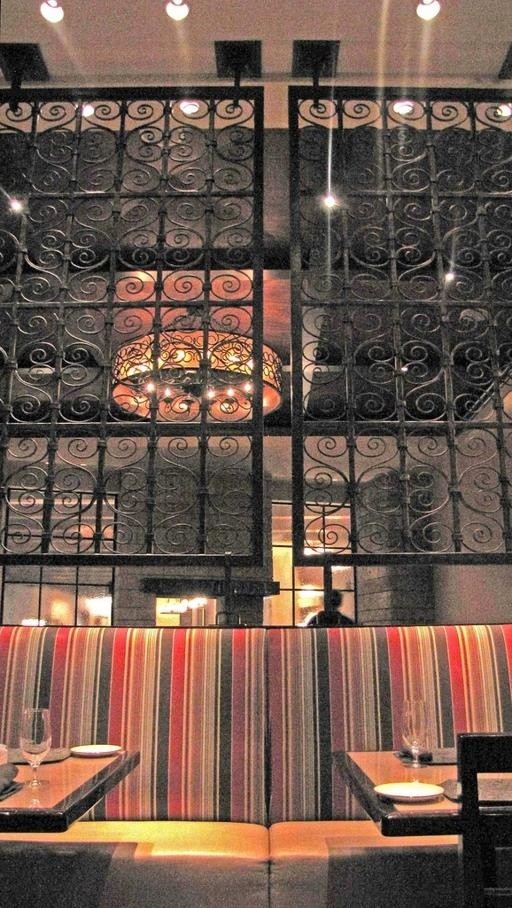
19;707;52;788
399;700;432;769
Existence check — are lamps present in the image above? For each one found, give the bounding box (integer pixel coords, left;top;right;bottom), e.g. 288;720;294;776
107;329;283;423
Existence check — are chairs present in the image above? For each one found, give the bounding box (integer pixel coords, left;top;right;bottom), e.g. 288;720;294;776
458;735;512;908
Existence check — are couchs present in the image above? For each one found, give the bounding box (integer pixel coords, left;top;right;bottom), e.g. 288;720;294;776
1;625;512;908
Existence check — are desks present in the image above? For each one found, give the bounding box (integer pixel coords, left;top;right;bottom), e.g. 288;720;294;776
3;741;142;831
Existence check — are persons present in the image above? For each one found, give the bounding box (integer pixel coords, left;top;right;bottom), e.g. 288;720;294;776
305;589;354;627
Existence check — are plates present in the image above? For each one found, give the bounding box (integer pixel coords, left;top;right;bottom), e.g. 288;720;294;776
70;744;123;758
373;781;445;803
